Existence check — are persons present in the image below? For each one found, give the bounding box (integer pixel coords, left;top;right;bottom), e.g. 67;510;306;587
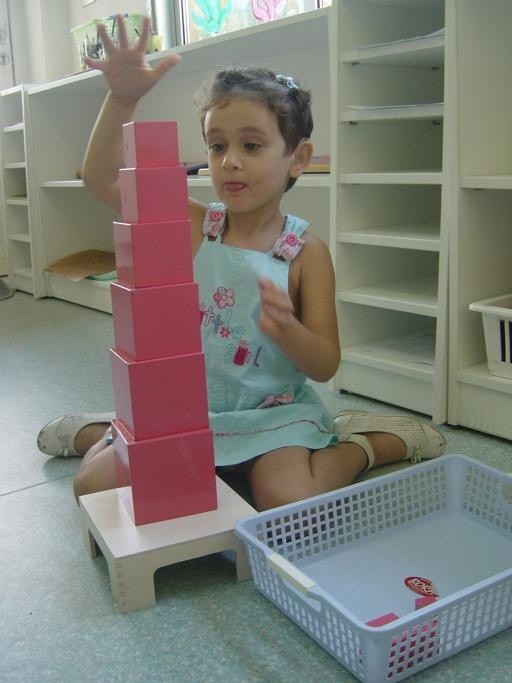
33;11;451;534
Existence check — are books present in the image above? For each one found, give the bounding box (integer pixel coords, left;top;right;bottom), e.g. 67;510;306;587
302;153;332;175
196;167;210;177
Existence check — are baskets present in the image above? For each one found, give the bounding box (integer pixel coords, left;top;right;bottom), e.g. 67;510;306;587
234;453;511;683
70;12;151;71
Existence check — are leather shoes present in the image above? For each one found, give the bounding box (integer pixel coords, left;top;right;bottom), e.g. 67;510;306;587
329;410;447;474
36;412;115;459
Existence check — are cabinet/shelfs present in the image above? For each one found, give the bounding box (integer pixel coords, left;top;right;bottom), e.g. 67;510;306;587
328;0;451;427
26;4;331;383
0;84;37;300
449;0;511;442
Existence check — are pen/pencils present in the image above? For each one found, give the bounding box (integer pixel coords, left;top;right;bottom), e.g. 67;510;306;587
78;18;153;68
